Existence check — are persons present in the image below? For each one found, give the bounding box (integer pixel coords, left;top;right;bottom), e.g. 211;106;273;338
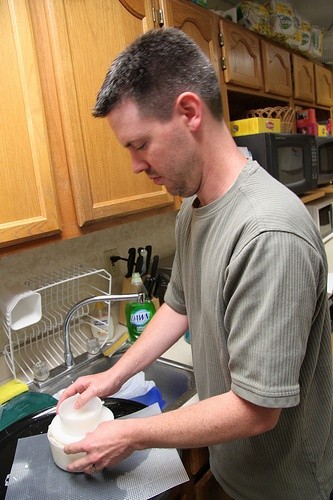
55;28;333;500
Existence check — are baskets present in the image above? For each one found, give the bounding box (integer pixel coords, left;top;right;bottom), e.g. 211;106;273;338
0;264;111;386
245;106;298;134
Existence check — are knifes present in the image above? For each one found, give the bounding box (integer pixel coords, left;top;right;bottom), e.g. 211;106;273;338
125;245;163;300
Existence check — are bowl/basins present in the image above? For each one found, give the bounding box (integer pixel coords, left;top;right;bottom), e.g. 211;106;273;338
47;405;114;472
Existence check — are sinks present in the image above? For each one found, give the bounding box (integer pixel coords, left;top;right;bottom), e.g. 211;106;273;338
32;343;201;421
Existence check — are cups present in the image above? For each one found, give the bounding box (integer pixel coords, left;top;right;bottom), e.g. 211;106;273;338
59;394;103;435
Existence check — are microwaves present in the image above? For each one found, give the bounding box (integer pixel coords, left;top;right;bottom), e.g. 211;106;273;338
320;136;333;183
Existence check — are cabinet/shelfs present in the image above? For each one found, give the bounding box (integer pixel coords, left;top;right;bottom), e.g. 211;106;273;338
166;0;333;139
0;0;181;257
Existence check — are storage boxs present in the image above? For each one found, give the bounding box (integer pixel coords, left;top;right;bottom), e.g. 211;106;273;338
231;117;281;137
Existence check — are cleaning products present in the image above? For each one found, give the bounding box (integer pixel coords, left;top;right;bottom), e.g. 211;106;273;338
124;272;156;343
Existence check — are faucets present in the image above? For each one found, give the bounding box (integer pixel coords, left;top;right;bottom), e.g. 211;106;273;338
63;292;146;367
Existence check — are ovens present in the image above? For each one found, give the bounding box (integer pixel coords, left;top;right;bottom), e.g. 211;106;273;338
233;134;319;195
